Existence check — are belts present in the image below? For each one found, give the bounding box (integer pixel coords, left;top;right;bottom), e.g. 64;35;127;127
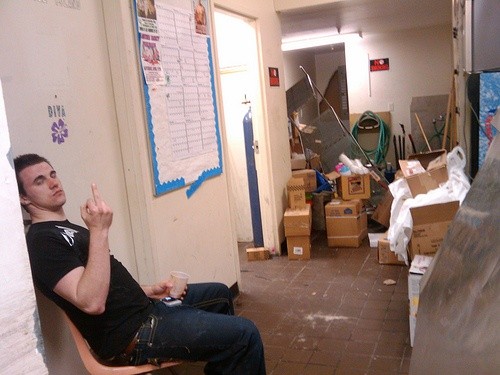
121;324;143;357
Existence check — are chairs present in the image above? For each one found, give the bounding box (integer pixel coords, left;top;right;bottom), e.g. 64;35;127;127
60;306;184;375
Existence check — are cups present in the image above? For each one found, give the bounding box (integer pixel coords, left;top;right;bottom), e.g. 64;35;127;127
169;271;189;299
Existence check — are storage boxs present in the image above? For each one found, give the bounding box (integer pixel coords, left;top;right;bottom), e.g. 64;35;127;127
377;231;408;264
246;247;270;260
397;149;459;347
367;232;383;248
284;111;375;260
370;187;394;228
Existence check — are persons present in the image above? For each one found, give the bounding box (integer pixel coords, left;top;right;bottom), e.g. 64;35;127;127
13;153;267;375
